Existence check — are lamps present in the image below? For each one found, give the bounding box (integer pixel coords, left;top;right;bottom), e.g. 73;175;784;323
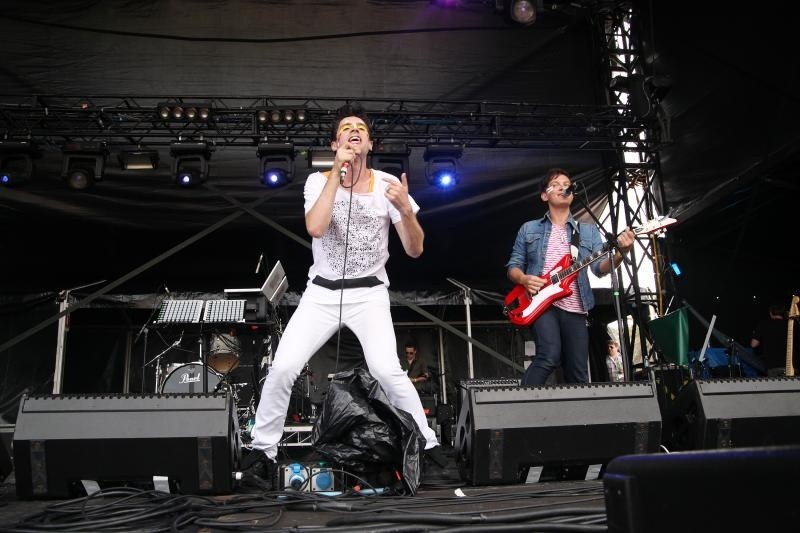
121;146;159;170
423;149;463;190
25;139;109;188
166;140;213;185
0;142;12;184
157;102;210;123
495;0;536;28
256;141;294;186
256;105;308;125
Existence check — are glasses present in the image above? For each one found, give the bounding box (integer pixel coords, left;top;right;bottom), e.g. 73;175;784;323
608;346;616;349
406;351;413;355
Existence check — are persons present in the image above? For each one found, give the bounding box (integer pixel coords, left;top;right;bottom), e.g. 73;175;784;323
605;340;625;384
506;169;633;385
751;304;789;356
248;104;441;459
400;344;429;383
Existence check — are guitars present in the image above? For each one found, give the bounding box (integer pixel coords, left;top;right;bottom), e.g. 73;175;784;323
784;295;800;376
502;215;678;324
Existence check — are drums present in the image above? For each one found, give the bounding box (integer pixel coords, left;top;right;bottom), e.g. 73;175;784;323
160;362;222;396
208;333;240;373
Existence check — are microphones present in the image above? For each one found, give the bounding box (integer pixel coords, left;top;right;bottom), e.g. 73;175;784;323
339;148;353;184
562;183;576;196
255;252;263;274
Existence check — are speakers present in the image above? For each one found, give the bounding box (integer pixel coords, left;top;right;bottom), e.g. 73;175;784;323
454;381;664;487
602;445;800;533
13;390;240;502
661;377;800;452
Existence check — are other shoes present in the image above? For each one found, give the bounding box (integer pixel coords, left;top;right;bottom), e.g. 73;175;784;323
237;449;273;492
421;446;450;480
